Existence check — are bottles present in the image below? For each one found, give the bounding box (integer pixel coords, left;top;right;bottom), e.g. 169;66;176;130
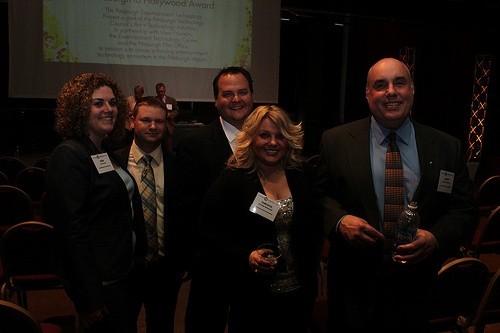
392;200;421;265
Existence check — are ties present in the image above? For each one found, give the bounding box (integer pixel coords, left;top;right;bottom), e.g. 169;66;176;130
383;132;404;260
140;154;158;262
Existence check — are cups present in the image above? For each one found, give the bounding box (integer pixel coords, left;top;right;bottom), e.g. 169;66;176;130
256;242;282;262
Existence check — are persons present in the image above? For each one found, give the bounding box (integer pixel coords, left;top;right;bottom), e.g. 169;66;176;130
45;72;148;333
155;83;179;158
111;96;191;333
173;67;255;333
304;58;481;333
121;85;145;140
199;105;326;333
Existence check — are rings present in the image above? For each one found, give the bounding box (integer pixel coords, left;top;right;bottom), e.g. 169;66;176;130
254;267;259;274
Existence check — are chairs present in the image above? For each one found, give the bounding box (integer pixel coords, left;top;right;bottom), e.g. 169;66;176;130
0;156;500;333
